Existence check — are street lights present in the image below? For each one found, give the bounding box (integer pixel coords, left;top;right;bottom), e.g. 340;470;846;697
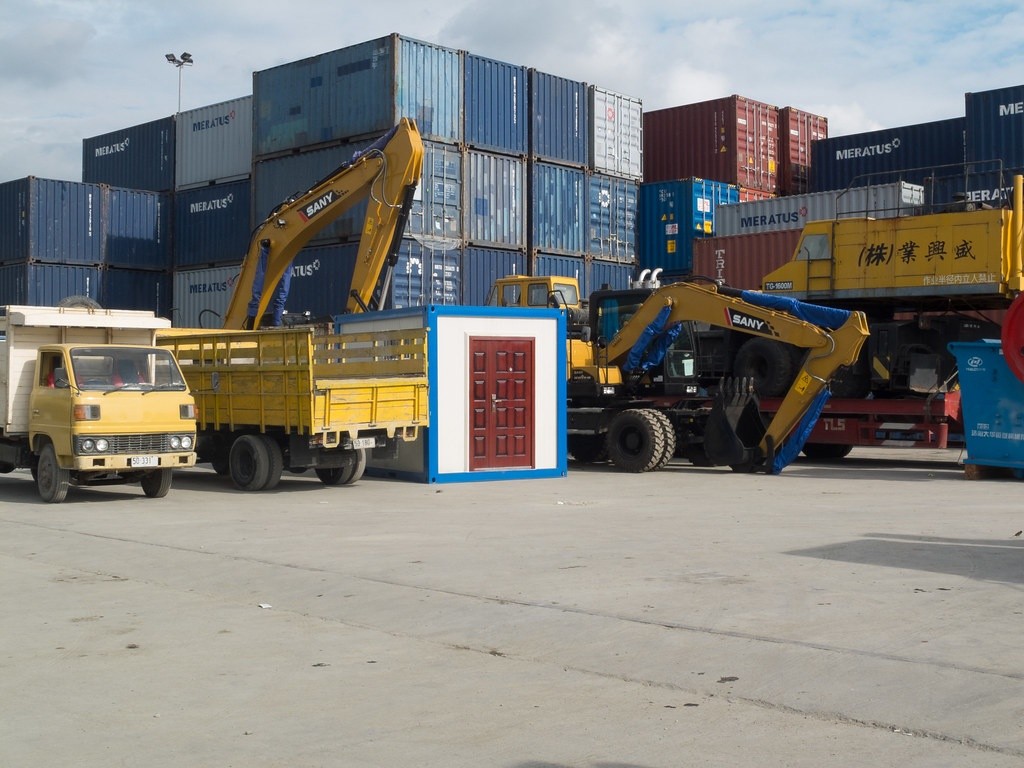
165;52;193;113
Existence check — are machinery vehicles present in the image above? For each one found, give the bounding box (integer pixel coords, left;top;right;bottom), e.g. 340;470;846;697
483;275;871;477
225;117;425;330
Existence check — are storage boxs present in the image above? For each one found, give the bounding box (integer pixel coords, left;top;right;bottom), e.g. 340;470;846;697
633;82;1024;291
946;338;1024;481
0;175;166;318
82;94;253;328
253;31;642;316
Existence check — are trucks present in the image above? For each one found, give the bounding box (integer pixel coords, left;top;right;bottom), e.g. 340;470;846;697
154;327;432;492
0;305;220;504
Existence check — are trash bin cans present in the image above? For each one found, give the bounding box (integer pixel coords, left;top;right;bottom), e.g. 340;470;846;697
945;340;1024;479
